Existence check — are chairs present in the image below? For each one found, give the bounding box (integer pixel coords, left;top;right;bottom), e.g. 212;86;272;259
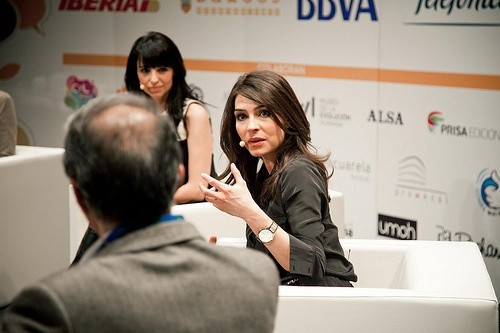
171;190;346;241
214;237;499;333
0;145;70;306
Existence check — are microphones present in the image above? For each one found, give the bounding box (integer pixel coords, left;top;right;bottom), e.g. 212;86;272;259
239;141;245;147
140;81;145;90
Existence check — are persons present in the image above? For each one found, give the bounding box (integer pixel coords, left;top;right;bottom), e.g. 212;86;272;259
0;93;279;333
70;30;218;267
199;70;357;287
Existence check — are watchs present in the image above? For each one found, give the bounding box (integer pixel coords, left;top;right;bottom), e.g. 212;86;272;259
255;221;278;244
